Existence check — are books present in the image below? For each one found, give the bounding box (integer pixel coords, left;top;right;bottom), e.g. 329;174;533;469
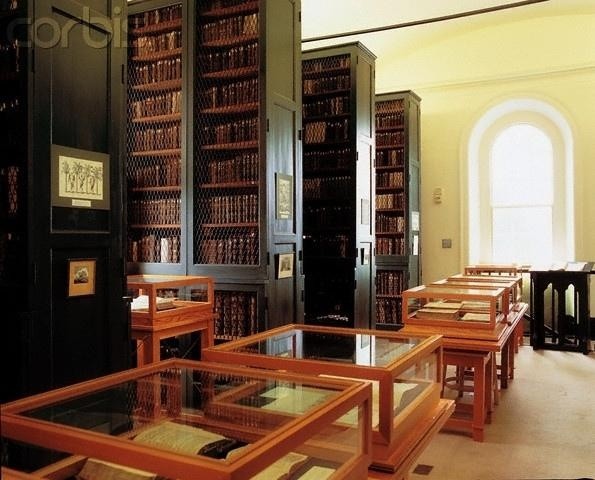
129;194;258;225
301;53;352;361
129;1;261;57
79;421;336;480
129;42;258;118
129;119;259;187
374;96;406;327
214;293;257;337
414;291;513;325
129;234;257;263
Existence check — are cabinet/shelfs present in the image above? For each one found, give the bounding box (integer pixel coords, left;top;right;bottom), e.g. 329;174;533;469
0;0;530;480
